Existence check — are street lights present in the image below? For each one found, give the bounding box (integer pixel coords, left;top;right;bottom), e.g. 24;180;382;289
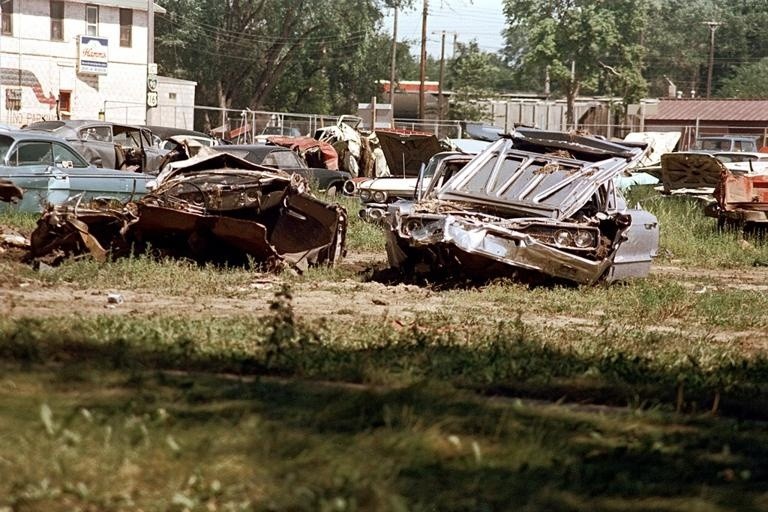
702;21;724;99
